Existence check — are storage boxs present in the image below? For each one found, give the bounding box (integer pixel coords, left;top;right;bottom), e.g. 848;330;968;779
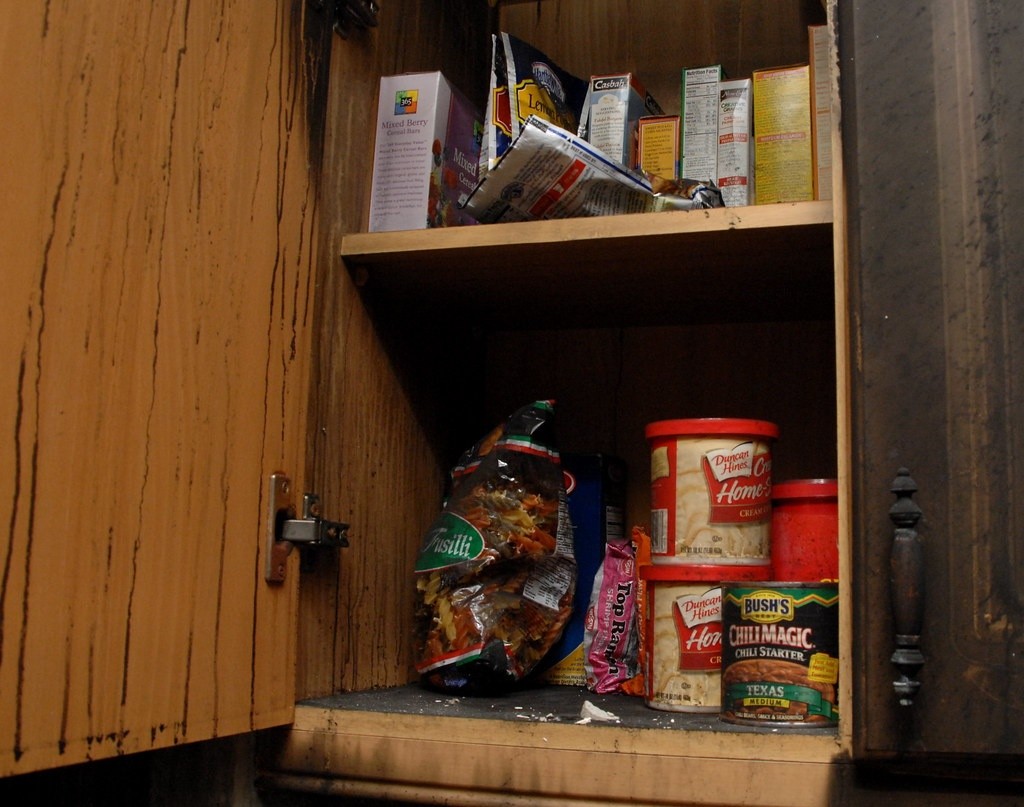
368;69;481;235
681;64;727;189
717;77;756;207
588;72;666;175
807;24;833;203
636;113;680;182
752;61;813;207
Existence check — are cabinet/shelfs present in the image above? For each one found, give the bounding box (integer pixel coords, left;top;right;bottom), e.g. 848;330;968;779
0;0;867;777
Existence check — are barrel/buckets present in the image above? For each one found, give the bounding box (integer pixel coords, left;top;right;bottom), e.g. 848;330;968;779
771;476;839;583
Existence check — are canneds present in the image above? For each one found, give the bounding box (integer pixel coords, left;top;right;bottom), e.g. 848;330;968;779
720;580;838;729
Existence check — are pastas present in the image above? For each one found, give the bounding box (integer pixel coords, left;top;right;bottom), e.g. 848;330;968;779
418;481;571;678
